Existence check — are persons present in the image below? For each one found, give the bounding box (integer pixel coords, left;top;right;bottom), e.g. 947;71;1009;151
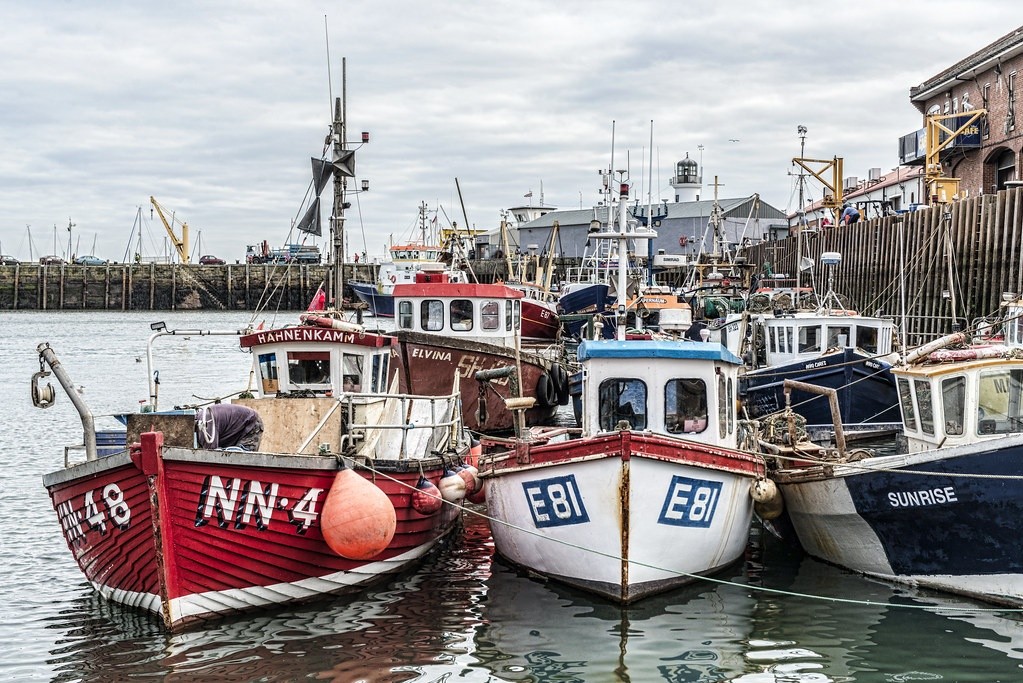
839;205;860;226
194;404;264;453
355;253;359;263
362;252;365;256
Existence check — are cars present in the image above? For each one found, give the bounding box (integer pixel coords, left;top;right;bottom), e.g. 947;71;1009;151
199;255;226;265
73;256;107;266
0;255;21;265
40;256;68;266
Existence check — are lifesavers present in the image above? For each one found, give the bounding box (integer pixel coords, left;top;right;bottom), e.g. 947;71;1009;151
679;235;688;246
832;309;857;316
928;343;1010;362
299;312;364;333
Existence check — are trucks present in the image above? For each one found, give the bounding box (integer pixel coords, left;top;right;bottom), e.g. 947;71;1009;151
246;239;321;264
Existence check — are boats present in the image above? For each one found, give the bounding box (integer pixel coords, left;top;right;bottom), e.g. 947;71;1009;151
28;53;1022;631
